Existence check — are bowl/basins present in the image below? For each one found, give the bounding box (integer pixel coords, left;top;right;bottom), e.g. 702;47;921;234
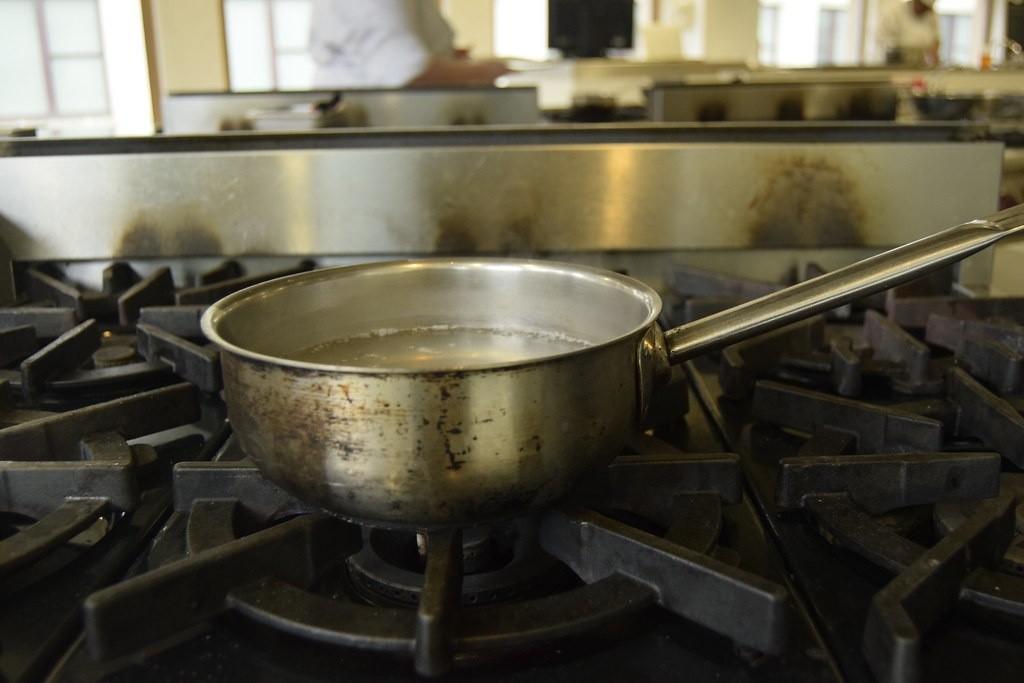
914;95;975;119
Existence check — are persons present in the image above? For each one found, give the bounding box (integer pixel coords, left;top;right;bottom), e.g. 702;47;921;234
307;0;525;91
878;0;941;67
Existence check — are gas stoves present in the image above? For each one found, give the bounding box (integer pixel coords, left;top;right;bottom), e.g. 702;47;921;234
0;121;1024;683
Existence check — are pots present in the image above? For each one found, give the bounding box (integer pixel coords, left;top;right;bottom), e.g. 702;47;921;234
198;202;1024;534
